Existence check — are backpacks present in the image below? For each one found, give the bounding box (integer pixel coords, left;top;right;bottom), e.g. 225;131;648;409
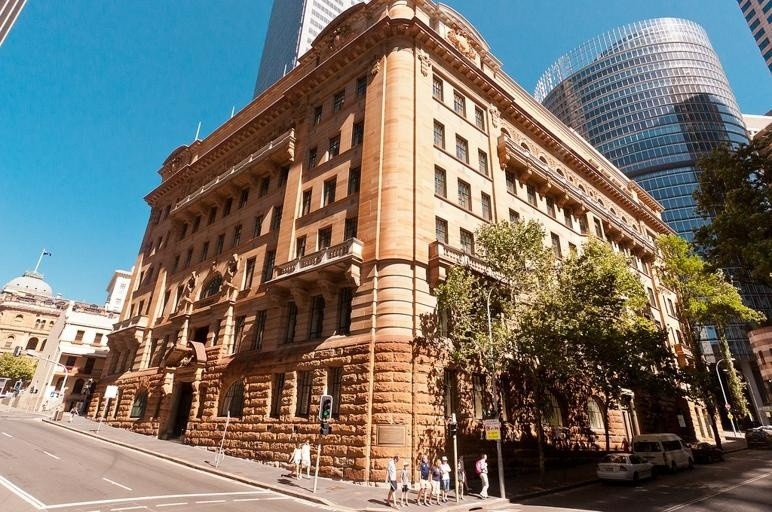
473;460;481;474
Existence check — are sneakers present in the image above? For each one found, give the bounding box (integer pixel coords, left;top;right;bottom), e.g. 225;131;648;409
384;498;448;509
479;492;489;500
288;474;312;481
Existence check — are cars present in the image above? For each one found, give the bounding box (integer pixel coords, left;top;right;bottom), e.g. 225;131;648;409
744;423;772;448
594;431;723;481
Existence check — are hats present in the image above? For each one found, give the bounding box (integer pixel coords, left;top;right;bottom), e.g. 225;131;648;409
442;456;448;461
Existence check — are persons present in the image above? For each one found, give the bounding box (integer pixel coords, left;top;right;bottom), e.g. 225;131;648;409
299;439;312;476
477;453;490;498
460;453;473;492
415;456;432;506
289;441;303;479
439;455;452;502
428;458;443;505
69;406;77;419
399;462;410;506
386;455;399;507
455;458;465;500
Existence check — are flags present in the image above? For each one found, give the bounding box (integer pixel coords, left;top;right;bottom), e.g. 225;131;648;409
44;250;53;256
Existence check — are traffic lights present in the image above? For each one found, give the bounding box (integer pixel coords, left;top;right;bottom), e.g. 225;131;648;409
321;396;333;420
16;381;21;388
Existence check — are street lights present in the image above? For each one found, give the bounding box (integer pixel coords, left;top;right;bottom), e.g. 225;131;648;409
487;261;542;500
713;356;737;440
